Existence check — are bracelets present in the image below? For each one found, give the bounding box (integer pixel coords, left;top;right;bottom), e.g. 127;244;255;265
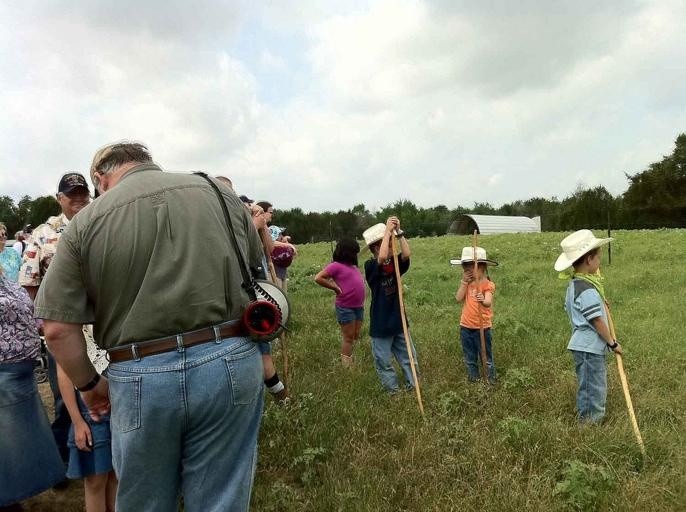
461;279;468;285
606;343;618;349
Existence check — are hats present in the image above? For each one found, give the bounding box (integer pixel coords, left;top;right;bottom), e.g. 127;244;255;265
58;172;89;195
450;246;499;268
360;222;397;255
553;228;613;272
239;195;256;204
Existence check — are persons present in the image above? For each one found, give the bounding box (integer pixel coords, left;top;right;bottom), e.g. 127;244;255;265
358;216;420;396
450;246;498;386
315;238;366;370
553;229;623;427
2;144;297;511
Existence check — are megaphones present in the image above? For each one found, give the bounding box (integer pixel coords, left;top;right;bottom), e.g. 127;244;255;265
243;279;291;343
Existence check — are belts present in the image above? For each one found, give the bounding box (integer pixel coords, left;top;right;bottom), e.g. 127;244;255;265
108;322;245;362
